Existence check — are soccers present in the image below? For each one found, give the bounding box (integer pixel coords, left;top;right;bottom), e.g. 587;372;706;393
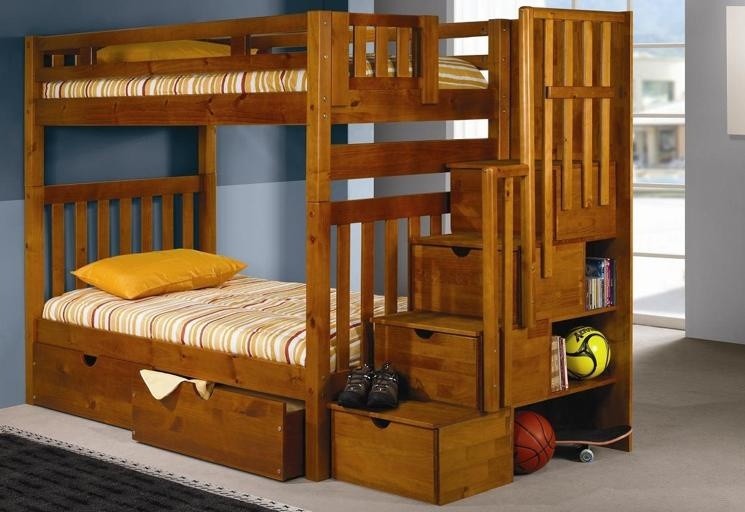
565;325;612;382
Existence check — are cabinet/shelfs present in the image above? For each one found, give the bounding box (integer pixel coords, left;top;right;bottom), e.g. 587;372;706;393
511;232;632;483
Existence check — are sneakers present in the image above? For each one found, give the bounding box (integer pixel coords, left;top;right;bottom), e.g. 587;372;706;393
335;364;375;412
365;365;402;414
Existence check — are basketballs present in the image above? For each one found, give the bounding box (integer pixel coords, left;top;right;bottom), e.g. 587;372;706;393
513;411;556;475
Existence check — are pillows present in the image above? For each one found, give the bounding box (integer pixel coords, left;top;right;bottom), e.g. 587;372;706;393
96;40;258;61
70;248;247;297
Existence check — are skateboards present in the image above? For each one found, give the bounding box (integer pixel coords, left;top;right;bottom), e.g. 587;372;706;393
556;423;633;462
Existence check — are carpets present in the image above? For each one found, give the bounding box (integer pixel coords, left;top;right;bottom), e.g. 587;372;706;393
1;426;310;512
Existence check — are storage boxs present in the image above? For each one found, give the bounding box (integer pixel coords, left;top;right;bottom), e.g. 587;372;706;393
331;413;509;506
402;233;518;322
374;323;495;412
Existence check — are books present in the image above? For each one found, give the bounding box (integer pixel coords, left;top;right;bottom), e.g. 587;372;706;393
584;256;617;311
551;335;569;391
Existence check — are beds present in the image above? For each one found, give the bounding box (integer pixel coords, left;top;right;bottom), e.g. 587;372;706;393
22;15;504;479
